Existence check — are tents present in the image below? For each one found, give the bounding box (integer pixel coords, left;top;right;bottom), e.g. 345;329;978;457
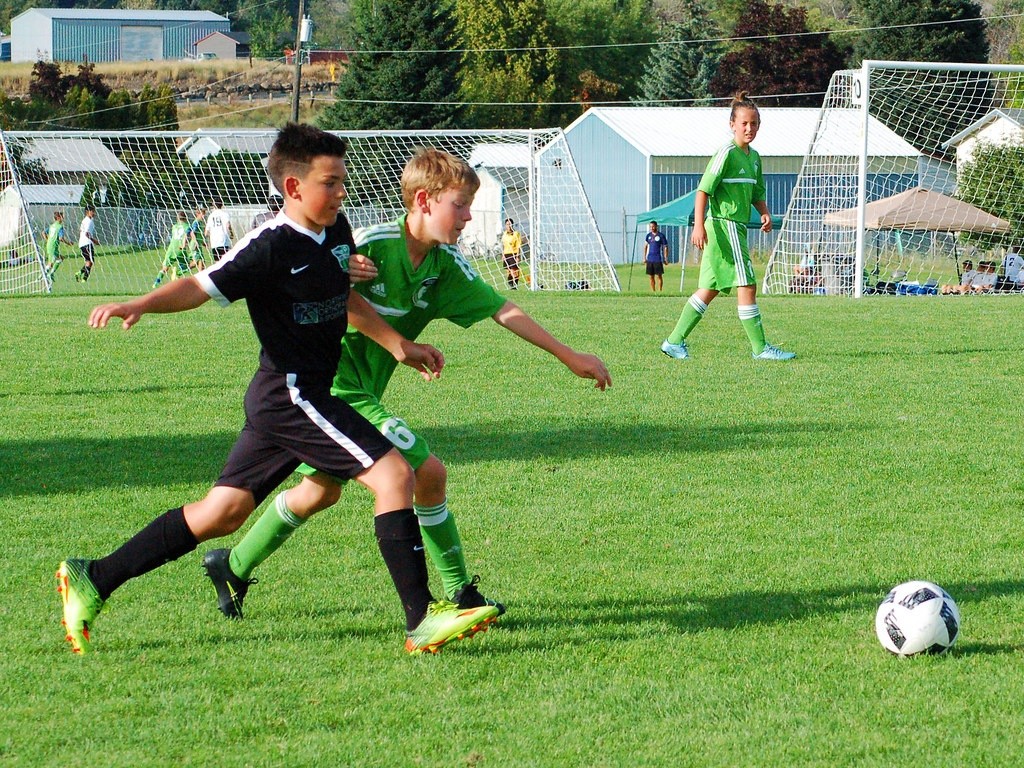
628;188;788;292
815;186;1011;285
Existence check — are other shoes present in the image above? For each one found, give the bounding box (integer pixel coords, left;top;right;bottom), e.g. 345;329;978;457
75;271;87;283
49;276;55;283
171;268;177;281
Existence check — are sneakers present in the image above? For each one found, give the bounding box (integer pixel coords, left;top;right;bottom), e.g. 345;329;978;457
54;557;104;655
201;549;259;620
753;342;795;363
405;598;500;656
661;339;689;359
449;576;505;617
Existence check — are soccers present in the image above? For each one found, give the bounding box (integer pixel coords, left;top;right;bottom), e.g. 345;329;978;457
875;580;960;658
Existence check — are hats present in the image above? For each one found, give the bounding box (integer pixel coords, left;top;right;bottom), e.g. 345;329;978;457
963;260;972;265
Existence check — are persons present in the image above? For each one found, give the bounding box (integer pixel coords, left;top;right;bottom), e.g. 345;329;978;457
34;212;73;282
55;121;499;655
9;250;18;266
942;260;998;295
74;204;100;283
205;198;233;262
1002;247;1024;283
501;218;521;289
643;221;668;291
171;207;209;281
252;195;284;230
661;89;796;360
152;212;191;289
201;150;613;620
